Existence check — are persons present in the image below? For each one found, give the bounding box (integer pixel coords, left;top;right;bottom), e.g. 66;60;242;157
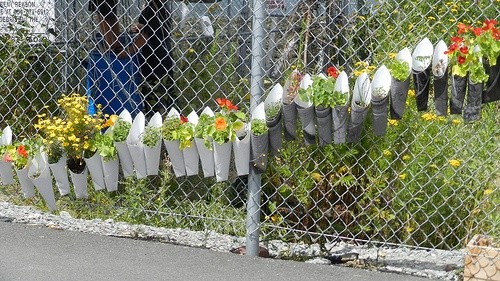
116;0;174;110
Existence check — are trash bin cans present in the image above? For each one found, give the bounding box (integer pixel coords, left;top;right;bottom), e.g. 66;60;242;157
87;30;144;134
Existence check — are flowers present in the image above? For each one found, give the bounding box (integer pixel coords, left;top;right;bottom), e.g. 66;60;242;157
310;66;339;108
473;18;500;67
31;134;47;177
206;97;238;144
83;104;117;157
37;114;63;164
3;141;30;169
445;22;472;76
287;63;305;102
58;93;91;173
172;114;195;150
